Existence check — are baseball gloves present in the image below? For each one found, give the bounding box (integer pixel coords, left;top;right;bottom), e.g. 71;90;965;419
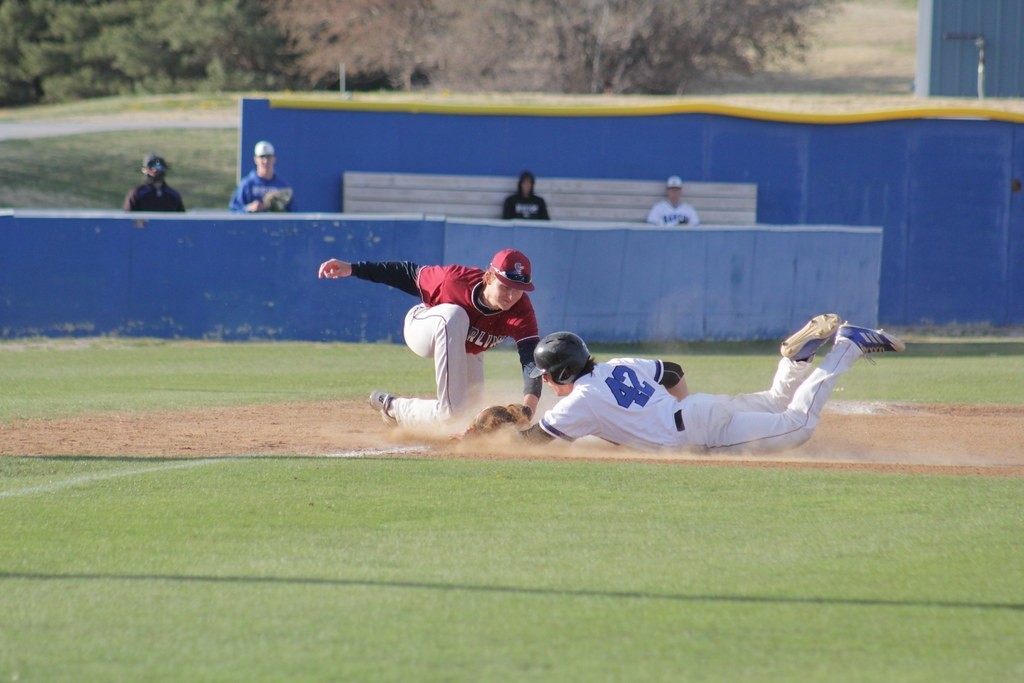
447;403;533;443
263;188;293;210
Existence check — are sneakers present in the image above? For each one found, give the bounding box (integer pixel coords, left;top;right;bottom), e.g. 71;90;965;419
780;314;841;361
835;321;905;366
369;392;395;424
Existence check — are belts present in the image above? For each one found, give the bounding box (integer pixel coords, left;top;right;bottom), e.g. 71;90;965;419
674;410;685;432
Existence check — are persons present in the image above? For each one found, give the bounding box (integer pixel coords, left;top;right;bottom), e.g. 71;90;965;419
501;171;551;221
513;312;907;457
647;174;701;227
228;139;298;213
318;246;542;438
122;153;186;212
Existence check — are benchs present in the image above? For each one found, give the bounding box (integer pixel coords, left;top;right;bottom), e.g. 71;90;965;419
342;172;757;225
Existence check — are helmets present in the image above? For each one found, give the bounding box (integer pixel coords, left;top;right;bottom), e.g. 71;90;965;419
530;332;590;385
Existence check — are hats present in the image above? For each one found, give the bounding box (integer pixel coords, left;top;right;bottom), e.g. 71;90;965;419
141;152;166;169
254;141;275;156
489;250;535;291
667;176;683;189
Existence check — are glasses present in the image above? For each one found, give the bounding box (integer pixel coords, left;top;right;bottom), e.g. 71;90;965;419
493;267;532;284
